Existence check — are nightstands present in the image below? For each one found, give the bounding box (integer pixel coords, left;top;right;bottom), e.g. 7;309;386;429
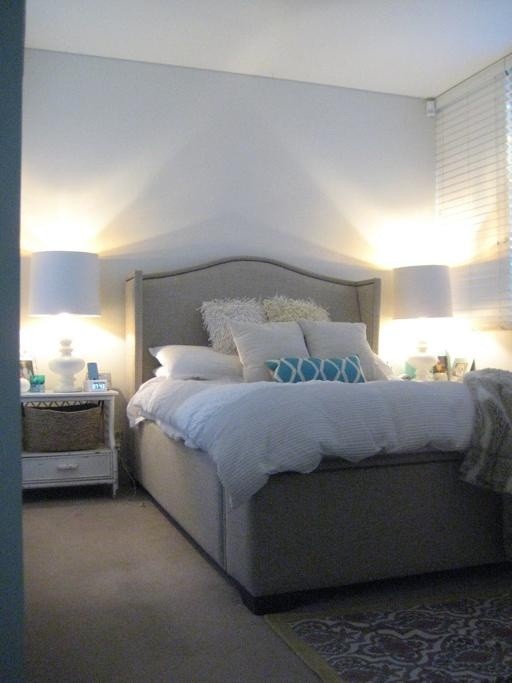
20;389;119;499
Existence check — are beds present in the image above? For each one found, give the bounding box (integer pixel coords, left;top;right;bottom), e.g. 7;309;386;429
122;257;511;615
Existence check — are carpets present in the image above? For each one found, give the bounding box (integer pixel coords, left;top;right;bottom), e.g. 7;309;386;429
263;578;512;683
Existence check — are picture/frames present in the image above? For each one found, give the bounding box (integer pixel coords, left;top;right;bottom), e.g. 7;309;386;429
448;358;473;384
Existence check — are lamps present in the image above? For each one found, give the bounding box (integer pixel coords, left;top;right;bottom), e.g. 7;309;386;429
392;265;454;381
28;251;101;392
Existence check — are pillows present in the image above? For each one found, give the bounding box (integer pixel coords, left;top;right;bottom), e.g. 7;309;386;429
153;367;239;383
262;295;331;337
227;318;311;383
265;352;368;383
298;319;390;382
197;298;268;354
149;345;242;381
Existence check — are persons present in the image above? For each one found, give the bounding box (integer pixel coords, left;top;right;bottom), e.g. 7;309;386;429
20;361;33;379
455;363;465;377
